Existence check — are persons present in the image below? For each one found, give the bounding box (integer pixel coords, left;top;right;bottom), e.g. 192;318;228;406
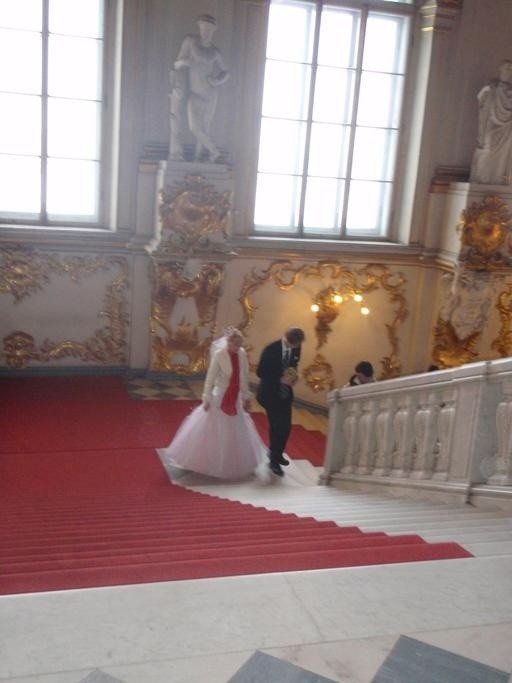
345;360;376;388
469;60;512;185
255;328;304;479
161;326;271;481
172;14;230;165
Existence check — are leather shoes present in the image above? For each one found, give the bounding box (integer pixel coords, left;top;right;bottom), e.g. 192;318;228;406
269;462;283;477
277;457;290;467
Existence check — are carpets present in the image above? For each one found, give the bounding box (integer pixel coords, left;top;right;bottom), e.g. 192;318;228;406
227;650;335;683
80;670;121;683
371;635;512;682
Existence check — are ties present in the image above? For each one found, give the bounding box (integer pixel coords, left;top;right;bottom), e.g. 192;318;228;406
281;350;289;372
